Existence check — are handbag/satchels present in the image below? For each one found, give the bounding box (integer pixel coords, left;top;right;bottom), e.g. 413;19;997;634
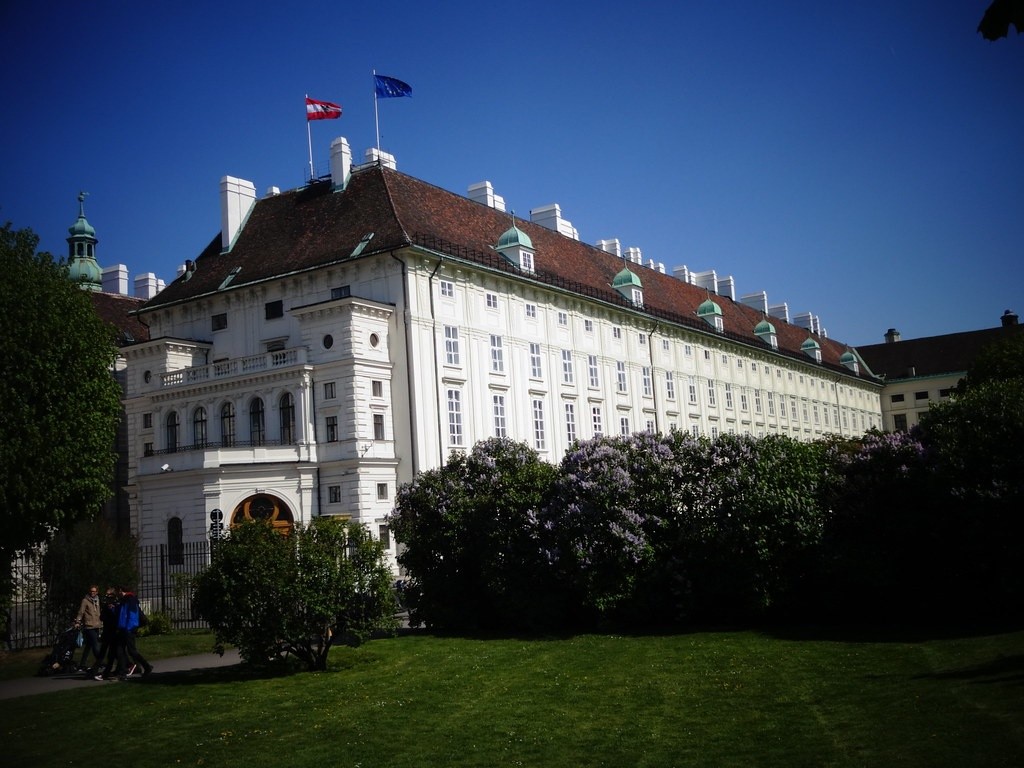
138;607;149;628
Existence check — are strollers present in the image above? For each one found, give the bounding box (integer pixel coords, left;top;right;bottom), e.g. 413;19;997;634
33;618;83;676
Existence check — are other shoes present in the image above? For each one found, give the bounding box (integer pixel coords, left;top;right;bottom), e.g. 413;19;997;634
78;669;83;672
125;663;136;677
94;675;103;680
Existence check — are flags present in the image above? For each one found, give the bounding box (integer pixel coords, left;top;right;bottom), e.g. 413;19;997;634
305;94;342;120
374;70;412;98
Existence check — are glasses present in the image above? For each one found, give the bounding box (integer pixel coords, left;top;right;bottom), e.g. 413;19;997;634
90;590;97;593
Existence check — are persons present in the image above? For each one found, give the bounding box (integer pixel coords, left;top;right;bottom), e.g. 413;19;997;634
75;585;154;682
